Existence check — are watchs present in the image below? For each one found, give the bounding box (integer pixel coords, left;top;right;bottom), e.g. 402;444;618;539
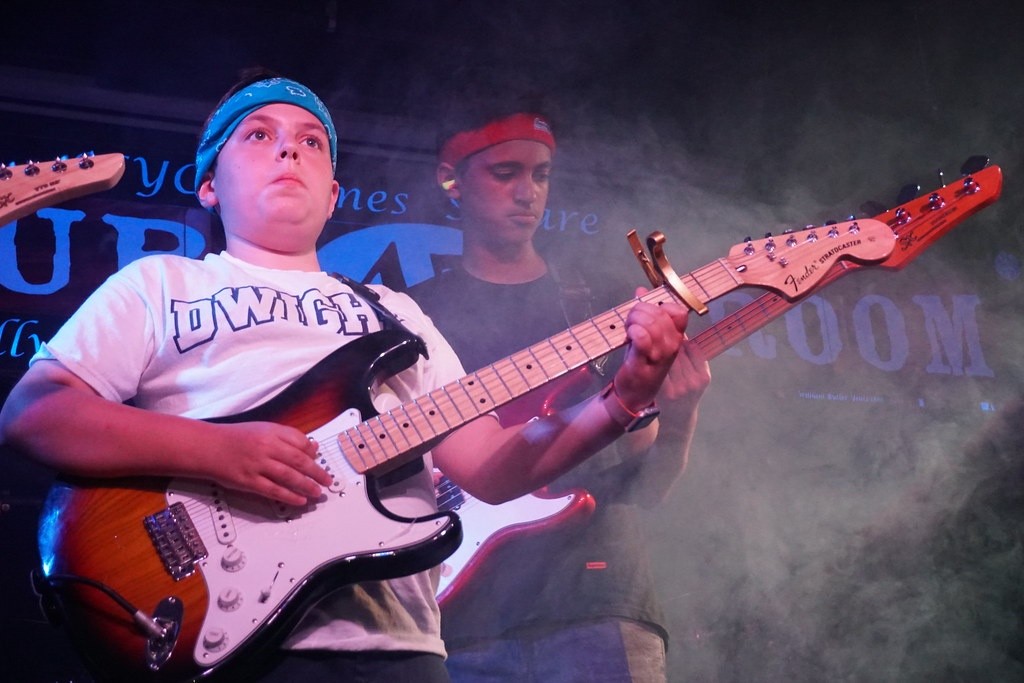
599;381;660;433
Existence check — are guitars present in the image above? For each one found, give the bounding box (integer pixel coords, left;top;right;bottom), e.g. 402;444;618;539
29;207;898;683
0;148;130;233
424;153;1009;647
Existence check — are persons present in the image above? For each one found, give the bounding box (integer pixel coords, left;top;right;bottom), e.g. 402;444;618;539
398;91;712;683
0;66;689;683
